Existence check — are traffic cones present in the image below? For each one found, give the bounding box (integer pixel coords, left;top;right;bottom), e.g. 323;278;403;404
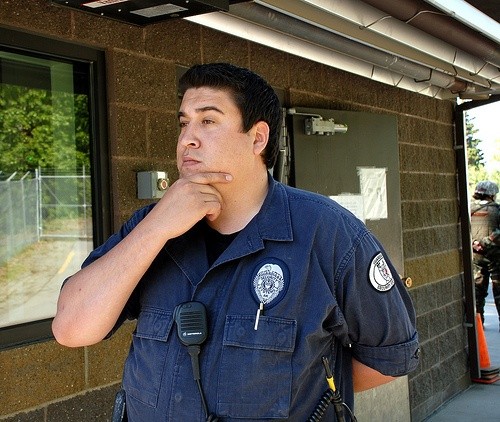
472;312;499;384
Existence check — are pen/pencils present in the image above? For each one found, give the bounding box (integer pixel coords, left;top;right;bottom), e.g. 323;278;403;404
254;303;265;331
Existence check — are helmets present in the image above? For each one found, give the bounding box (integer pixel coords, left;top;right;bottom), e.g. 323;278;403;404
475;182;498;195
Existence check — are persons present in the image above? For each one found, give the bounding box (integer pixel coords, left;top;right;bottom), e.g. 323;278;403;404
470;180;500;333
51;62;421;421
